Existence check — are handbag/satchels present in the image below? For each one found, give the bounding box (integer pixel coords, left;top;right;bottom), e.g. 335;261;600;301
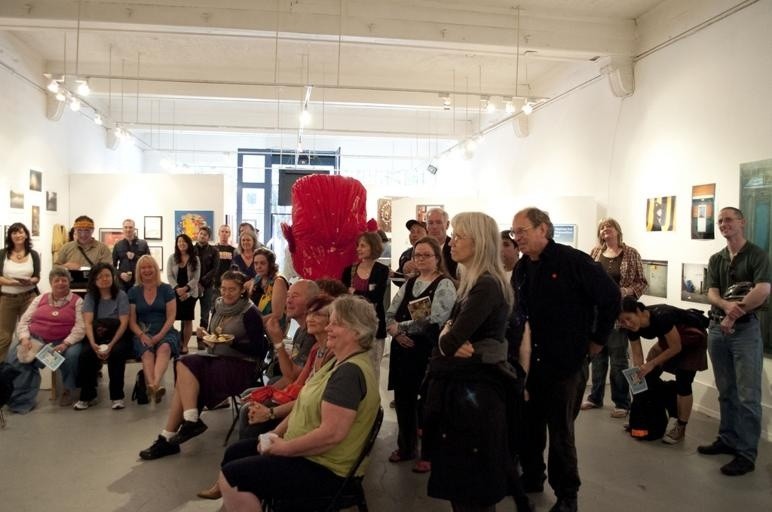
132;369;148;405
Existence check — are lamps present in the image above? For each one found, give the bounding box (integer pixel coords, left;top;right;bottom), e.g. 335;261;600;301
47;76;108;126
436;87;534;117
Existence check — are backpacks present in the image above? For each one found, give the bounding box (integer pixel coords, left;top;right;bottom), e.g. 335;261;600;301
627;382;668;441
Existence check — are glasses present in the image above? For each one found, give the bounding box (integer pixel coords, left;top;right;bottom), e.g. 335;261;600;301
449;231;472;242
508;226;532;240
413;253;435;260
304;310;328;319
218;287;236;293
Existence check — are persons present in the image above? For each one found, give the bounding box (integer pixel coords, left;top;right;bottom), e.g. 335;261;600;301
576;219;647;418
0;215;179;410
695;206;771;473
139;209;619;511
615;296;707;445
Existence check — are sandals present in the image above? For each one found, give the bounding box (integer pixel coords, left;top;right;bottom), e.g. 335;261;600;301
412;461;431;472
147;384;157;399
389;449;401;463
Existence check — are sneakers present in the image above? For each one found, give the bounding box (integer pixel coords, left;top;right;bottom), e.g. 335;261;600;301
60;392;75;406
111;399;125;409
170;418;208;446
139;434;180;460
73;396;98;410
662;423;686;444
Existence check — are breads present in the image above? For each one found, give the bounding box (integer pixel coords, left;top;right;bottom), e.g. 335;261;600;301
206;333;231;342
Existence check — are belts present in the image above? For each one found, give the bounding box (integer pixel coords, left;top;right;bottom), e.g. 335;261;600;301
1;289;35;298
708;314;756;324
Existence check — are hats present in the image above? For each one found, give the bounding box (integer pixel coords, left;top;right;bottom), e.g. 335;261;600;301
405;219;427;231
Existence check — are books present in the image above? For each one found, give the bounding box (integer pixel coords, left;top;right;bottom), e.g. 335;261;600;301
621;366;649;395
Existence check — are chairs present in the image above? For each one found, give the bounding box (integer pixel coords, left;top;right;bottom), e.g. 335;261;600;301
224;333;270;447
262;403;384;511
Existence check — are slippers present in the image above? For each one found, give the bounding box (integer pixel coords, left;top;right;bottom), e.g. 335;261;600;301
610;408;629;417
580;401;600;410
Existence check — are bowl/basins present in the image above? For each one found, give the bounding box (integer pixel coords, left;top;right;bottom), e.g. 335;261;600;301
202;333;234;344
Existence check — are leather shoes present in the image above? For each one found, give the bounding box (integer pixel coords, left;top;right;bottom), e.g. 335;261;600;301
697;436;736;455
197;490;222;499
519;476;544;493
549;499;577;512
720;455;755;476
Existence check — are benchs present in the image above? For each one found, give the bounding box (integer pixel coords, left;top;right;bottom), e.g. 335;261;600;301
52;356;159;405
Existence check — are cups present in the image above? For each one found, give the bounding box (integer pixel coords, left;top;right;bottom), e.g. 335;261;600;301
260;432;279;450
98;343;108;360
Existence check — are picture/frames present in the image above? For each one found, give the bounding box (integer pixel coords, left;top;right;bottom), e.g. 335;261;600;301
99;228;139;250
144;216;163;240
148;245;164;271
